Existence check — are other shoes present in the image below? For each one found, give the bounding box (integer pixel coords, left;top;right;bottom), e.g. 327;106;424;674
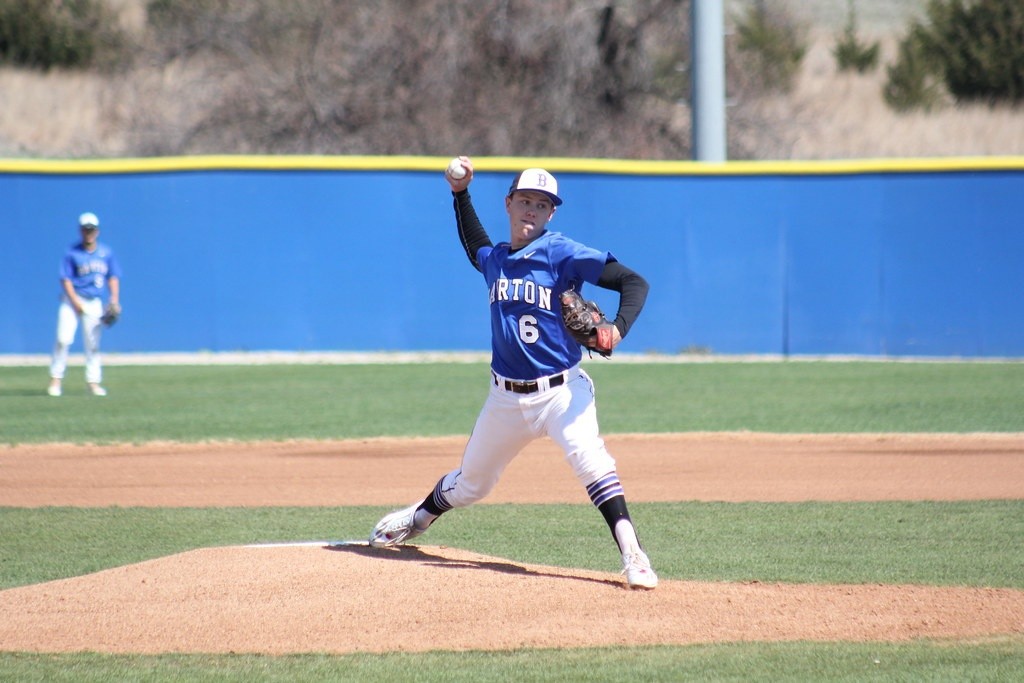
87;385;106;396
47;385;61;396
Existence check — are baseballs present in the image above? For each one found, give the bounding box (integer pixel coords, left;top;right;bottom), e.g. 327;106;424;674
448;157;467;179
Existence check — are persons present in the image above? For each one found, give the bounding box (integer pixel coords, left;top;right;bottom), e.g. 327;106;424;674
48;212;120;397
367;156;658;589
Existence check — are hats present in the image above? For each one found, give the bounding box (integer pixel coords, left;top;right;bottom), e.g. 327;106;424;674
79;213;98;229
509;168;563;206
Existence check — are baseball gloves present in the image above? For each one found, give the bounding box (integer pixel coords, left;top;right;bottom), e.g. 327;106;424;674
99;306;121;328
559;288;614;356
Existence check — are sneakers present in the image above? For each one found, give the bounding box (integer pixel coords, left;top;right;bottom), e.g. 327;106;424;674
368;500;427;546
622;544;658;588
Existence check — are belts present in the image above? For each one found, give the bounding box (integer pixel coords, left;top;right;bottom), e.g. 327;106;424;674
491;370;563;393
